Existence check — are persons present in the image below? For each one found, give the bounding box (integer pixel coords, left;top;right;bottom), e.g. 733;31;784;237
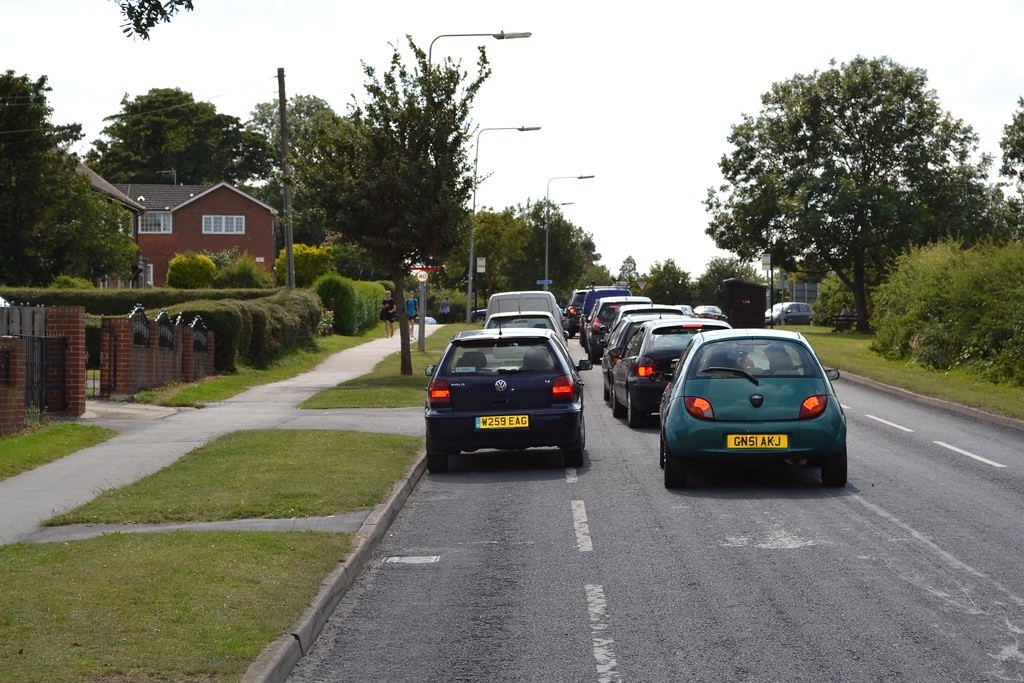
440;297;451;324
405;291;419;341
837;302;851;331
382;290;396;338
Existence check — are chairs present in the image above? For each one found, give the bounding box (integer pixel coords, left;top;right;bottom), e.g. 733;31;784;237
455;357;481;372
463;351;493;372
699;352;747;377
767;351;801;376
518;349;555;371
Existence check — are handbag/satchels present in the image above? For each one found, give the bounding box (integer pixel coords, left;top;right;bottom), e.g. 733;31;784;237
380;308;385;321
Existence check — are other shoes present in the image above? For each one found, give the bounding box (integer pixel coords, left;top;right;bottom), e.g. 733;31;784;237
386;336;389;338
391;331;394;337
409;331;413;337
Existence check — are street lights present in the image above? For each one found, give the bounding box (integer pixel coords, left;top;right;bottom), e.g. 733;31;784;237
464;125;541;324
416;29;534;354
544;173;595;293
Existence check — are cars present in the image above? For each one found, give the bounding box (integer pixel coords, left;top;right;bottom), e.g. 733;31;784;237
657;327;850;489
424;327;595;475
467;275;815;427
